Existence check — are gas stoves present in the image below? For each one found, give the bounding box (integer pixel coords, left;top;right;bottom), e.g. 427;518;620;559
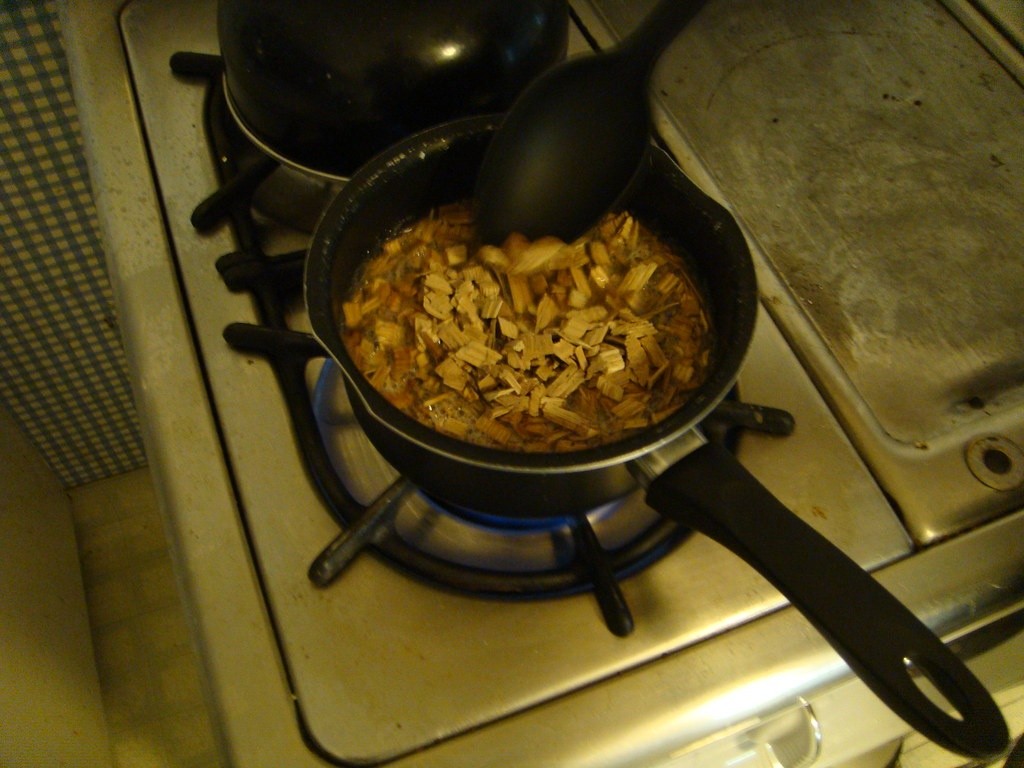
113;1;1024;765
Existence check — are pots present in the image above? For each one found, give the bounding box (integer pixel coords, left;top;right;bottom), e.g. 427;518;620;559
213;0;572;236
305;114;1009;760
